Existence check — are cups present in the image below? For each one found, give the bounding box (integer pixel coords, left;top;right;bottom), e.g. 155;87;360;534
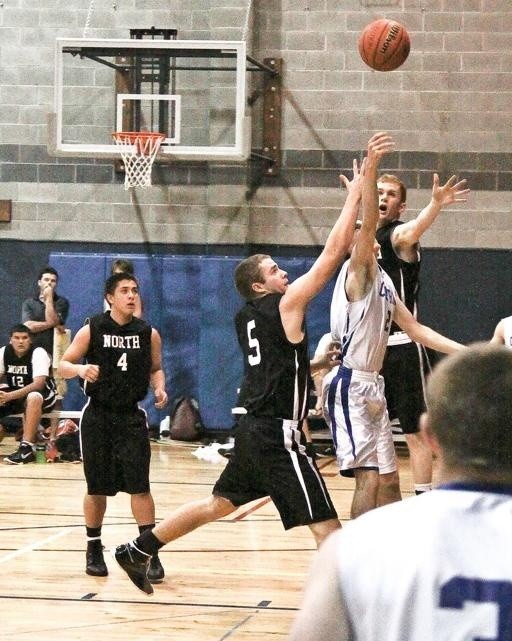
36;447;46;464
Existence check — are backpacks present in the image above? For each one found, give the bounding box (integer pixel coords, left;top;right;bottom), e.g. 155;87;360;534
170;396;202;441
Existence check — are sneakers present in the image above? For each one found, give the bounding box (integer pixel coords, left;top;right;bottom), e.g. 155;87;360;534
3;445;36;464
87;539;107;576
115;541;164;597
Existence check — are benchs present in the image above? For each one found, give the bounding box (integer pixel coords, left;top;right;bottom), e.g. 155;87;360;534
6;410;84;441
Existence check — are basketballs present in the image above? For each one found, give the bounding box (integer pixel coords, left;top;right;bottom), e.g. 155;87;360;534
358;19;410;71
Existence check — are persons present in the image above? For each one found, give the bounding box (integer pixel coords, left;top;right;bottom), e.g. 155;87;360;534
115;157;367;594
488;315;511;350
2;324;58;465
45;326;71;463
310;333;336;417
59;273;167;583
103;259;142;319
286;343;512;641
373;172;470;495
321;133;511;521
21;266;69;441
